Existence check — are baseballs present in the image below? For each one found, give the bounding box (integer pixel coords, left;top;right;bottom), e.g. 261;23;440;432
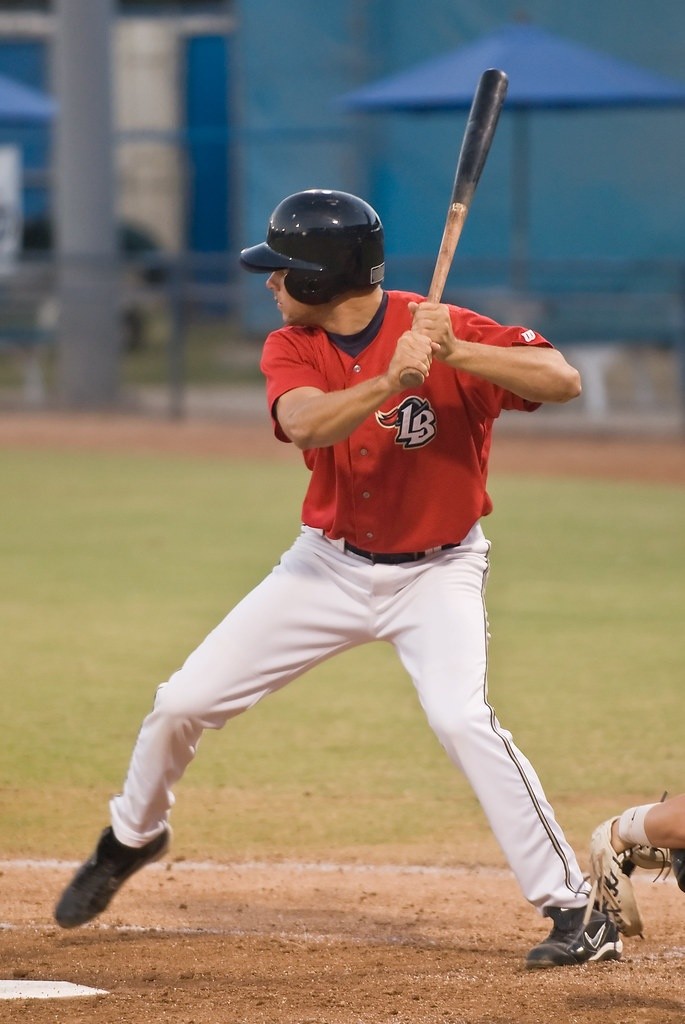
398;67;509;389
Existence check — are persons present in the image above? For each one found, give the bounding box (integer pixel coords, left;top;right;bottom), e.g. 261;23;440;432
588;793;685;938
51;189;622;972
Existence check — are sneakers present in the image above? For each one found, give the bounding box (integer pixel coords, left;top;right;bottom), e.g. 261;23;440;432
524;894;624;969
52;819;175;930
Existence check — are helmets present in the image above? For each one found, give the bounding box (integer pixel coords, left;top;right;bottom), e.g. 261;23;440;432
237;188;386;304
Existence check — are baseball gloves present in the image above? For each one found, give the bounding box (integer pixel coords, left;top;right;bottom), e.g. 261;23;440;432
589;815;672;938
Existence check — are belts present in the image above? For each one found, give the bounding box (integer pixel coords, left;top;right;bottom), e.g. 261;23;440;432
344;542;460;565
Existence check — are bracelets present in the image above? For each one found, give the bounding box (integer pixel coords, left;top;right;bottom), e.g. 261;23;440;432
619;801;661;848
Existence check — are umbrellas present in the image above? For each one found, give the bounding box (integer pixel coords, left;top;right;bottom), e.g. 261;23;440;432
0;75;57;128
338;12;685;241
